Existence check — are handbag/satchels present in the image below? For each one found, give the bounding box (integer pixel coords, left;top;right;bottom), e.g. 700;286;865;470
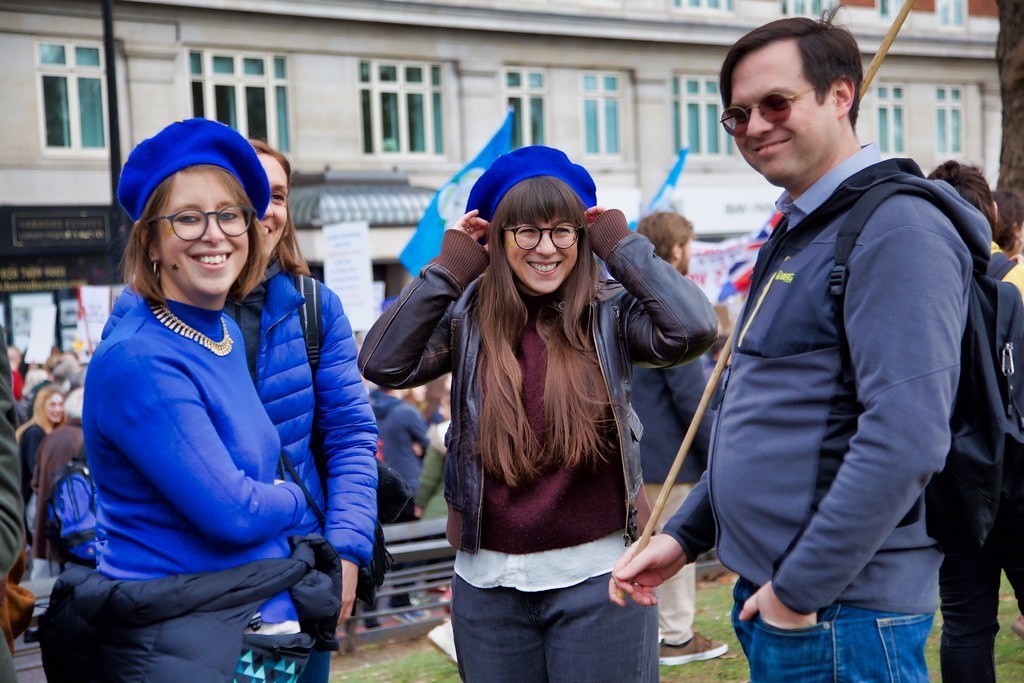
352;520;395;604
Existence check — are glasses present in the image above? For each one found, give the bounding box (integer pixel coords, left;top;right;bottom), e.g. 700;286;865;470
496;223;585;250
719;78;829;139
145;204;259;242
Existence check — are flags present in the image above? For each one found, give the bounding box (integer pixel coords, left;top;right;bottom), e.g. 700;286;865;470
399;110;514;279
628;148;688;231
717;210;782;300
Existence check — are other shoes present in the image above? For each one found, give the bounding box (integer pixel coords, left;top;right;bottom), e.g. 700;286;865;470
428;617;459;666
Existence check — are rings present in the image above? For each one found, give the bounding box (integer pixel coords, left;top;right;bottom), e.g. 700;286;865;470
631;582;643;588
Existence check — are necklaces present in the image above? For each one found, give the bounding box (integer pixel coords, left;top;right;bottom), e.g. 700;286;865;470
150;304;234;356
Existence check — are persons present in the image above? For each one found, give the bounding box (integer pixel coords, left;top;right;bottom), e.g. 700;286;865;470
627;211;727;666
609;18;1024;683
354;329;458;666
355;145;719;683
1;324;84;683
82;118;307;683
102;139;372;683
928;159;1024;683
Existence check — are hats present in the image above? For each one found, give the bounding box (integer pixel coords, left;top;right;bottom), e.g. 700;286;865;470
116;118;271;221
465;145;598;247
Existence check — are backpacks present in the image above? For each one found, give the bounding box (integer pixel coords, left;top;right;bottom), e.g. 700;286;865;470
834;178;1024;555
46;462;99;568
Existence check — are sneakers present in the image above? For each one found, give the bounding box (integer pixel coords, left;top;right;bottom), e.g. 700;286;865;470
658;629;728;666
